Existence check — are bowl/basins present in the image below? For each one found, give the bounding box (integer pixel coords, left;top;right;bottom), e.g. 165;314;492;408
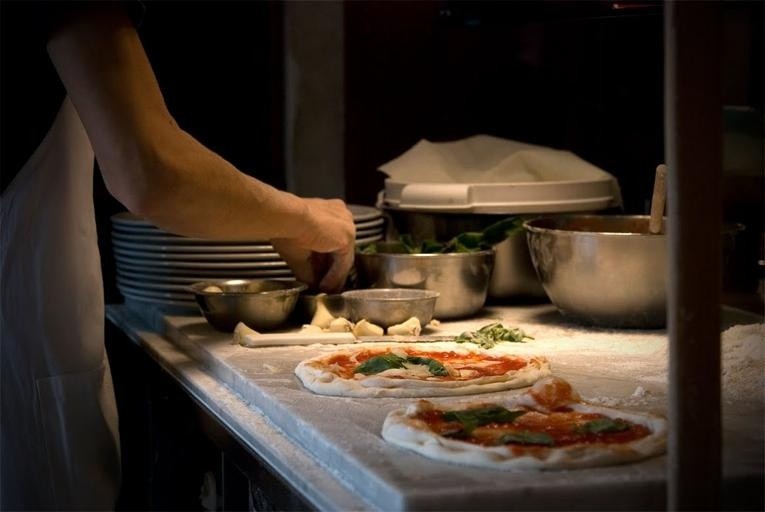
341;288;438;332
352;249;497;321
187;278;306;332
521;214;672;330
379;204;619;305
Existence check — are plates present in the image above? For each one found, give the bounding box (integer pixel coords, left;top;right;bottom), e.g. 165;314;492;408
111;203;385;313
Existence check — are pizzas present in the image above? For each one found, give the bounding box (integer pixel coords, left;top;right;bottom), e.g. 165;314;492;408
380;375;670;471
293;341;553;399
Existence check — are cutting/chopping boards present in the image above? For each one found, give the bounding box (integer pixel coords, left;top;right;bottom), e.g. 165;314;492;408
102;306;670;509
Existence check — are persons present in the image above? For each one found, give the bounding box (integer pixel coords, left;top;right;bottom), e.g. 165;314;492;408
0;0;356;512
